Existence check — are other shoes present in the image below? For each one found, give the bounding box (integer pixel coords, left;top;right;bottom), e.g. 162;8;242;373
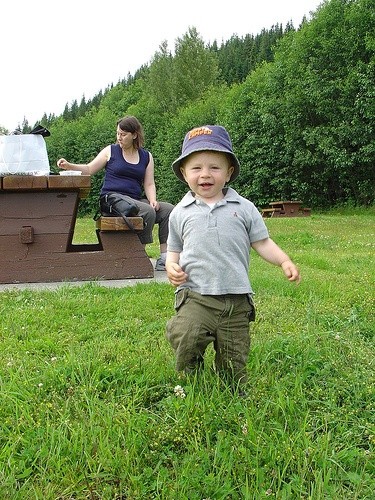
185;360;205;376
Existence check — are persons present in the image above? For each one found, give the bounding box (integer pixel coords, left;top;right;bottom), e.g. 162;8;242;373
164;124;300;399
57;116;174;272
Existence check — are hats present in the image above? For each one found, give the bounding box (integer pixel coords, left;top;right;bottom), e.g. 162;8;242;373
172;125;240;186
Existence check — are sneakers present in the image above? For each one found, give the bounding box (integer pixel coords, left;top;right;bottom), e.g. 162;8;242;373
155;258;166;271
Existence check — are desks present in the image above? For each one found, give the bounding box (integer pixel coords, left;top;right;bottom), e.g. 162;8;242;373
2;174;91;282
269;201;303;218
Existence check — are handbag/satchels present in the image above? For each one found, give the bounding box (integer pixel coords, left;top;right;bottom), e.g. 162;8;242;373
0;132;51;177
93;193;146;234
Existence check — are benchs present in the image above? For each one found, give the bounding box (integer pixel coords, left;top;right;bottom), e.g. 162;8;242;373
300;208;311;216
96;217;145;278
262;208;281;218
